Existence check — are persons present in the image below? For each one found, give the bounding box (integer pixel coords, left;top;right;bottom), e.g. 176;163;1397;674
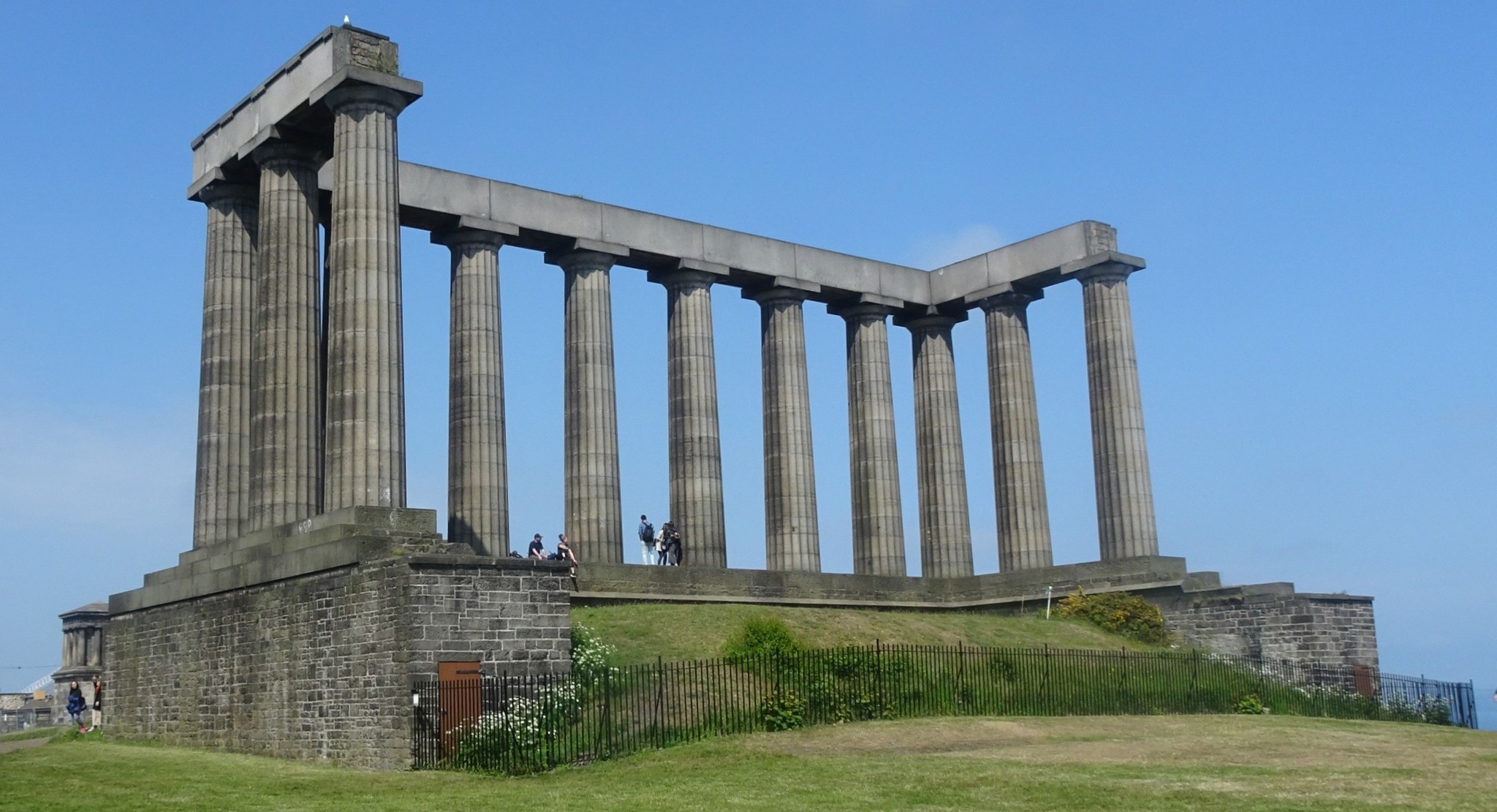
66;680;87;733
88;674;102;732
664;521;680;567
529;534;548;560
655;523;668;565
557;534;578;578
638;515;655;565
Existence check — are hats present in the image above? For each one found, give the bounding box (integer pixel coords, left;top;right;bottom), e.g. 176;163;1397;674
534;533;543;538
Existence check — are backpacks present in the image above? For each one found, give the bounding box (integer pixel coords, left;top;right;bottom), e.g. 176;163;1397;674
642;523;654;541
672;529;680;540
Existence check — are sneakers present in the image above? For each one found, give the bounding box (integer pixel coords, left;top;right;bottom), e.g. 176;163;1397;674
571;571;578;578
